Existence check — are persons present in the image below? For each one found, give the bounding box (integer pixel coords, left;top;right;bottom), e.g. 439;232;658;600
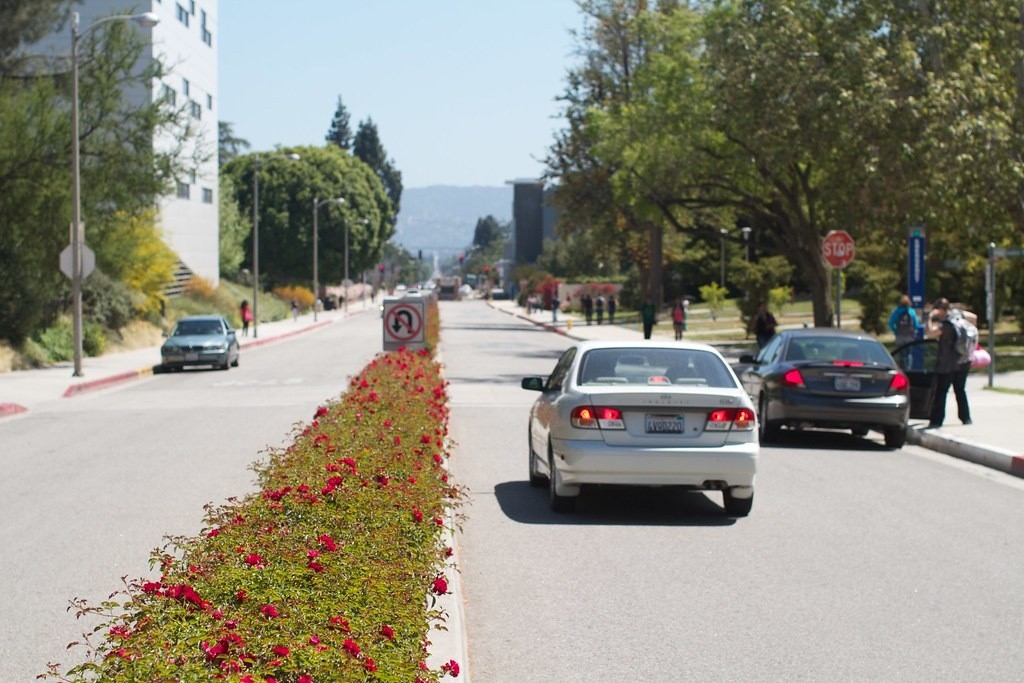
290;300;298;322
641;295;656;339
240;300;253;336
526;294;571;313
924;299;979;429
889;296;920;371
749;301;778;349
672;298;689;340
323;295;343;311
580;292;616;325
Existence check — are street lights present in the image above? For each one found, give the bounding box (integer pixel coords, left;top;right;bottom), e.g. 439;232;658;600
253;153;301;340
70;11;160;377
343;220;372;318
312;196;345;323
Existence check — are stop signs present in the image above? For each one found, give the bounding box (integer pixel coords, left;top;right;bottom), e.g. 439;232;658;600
823;230;854;269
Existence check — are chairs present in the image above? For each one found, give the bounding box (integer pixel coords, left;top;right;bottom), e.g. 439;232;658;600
597;377;628;384
842;347;864;361
677;378;706;384
785;343;805;361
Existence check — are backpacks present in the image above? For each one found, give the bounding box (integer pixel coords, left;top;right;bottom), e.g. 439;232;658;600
896;312;914;336
951;317;979;364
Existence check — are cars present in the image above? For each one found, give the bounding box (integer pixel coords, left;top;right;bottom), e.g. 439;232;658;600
160;316;241;372
739;327;939;450
521;341;759;517
